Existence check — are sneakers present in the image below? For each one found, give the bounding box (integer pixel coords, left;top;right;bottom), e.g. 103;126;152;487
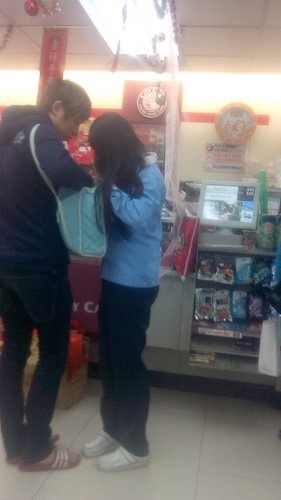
83;431;118;458
96;446;150;471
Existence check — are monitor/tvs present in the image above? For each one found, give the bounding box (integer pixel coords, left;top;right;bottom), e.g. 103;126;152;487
197;180;259;235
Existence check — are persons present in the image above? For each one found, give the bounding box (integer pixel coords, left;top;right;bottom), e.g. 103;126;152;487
82;112;165;471
0;78;95;474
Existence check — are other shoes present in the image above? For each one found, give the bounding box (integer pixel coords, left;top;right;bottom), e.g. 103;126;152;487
19;446;81;472
6;433;61;464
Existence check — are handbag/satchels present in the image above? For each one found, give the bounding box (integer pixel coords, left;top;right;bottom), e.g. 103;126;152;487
158;198;201;281
258;318;281;377
56;184;109;258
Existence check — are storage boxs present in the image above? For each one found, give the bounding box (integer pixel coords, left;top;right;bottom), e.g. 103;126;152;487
21;337;92;411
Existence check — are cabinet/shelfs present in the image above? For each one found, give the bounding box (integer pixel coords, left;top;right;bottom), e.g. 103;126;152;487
179;245;281;386
